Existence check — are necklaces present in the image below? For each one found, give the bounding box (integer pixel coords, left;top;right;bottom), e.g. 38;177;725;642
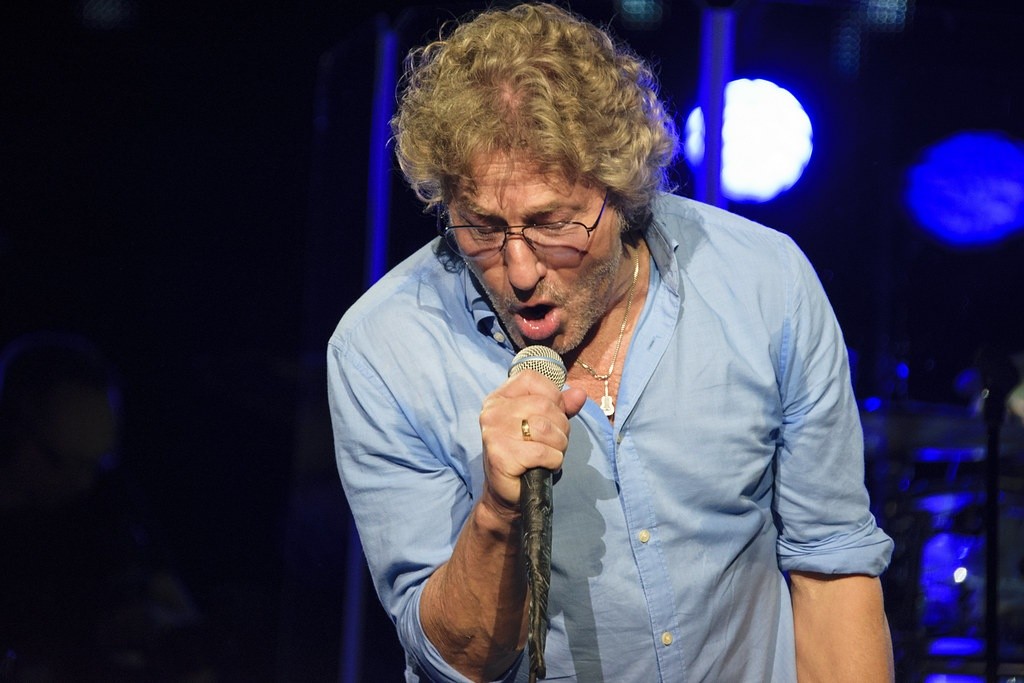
575;243;641;415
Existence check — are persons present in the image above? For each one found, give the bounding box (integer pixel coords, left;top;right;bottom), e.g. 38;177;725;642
328;3;896;683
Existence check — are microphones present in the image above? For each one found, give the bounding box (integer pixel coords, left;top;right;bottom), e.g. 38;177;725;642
507;344;568;604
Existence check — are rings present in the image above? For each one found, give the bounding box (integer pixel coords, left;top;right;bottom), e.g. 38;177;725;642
521;419;533;441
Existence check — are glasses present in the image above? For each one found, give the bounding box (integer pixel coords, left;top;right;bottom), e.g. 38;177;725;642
443;185;610;261
